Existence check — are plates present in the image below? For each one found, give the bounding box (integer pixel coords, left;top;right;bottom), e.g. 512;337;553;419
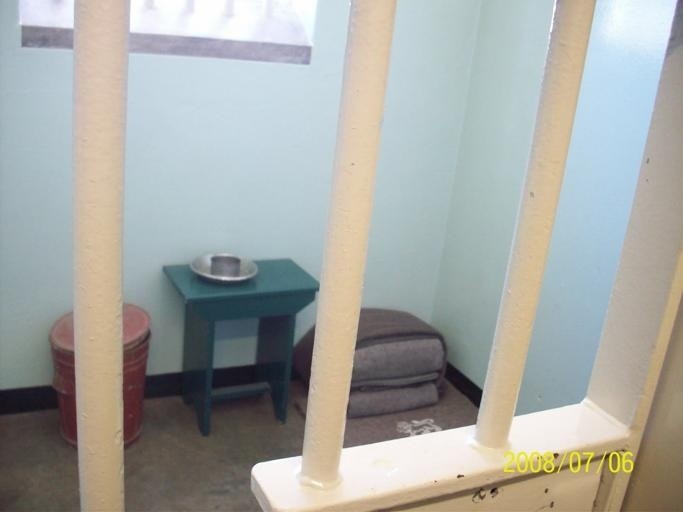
190;252;257;284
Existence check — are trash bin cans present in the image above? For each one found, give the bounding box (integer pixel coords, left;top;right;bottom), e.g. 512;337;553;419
47;302;153;450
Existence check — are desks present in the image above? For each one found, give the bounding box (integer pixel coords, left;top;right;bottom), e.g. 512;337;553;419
163;259;319;436
